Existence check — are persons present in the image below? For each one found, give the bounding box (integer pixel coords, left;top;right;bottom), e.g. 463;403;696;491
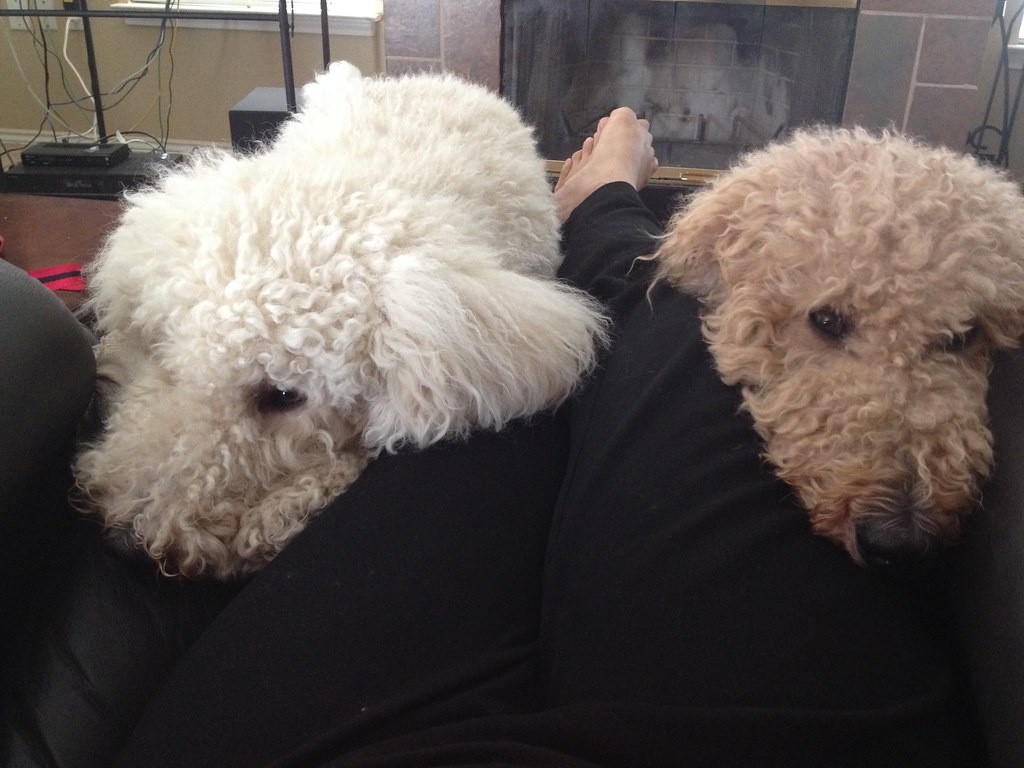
106;105;927;768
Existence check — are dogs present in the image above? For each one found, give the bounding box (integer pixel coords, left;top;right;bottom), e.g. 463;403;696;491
71;59;610;583
625;120;1024;571
